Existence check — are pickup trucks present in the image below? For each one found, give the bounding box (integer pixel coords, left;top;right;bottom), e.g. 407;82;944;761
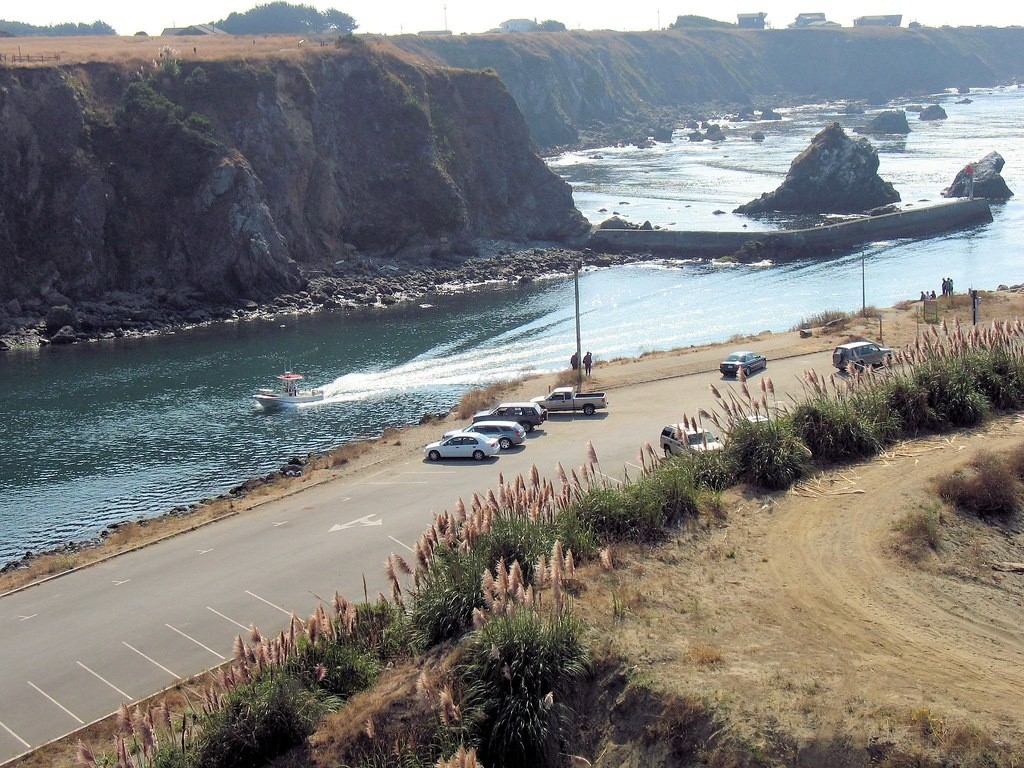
530;387;610;416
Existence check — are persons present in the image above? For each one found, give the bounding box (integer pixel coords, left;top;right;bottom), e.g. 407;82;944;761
286;382;297;397
920;291;936;301
583;351;592;376
942;278;953;297
571;352;578;370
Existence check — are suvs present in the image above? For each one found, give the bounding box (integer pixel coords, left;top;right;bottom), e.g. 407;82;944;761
441;421;527;450
832;341;894;372
659;422;725;458
471;402;548;434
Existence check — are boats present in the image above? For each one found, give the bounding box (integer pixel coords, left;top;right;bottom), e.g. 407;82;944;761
250;368;325;411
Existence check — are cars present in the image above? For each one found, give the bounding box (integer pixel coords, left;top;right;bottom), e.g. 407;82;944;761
746;415;768;423
423;431;501;462
719;351;767;378
